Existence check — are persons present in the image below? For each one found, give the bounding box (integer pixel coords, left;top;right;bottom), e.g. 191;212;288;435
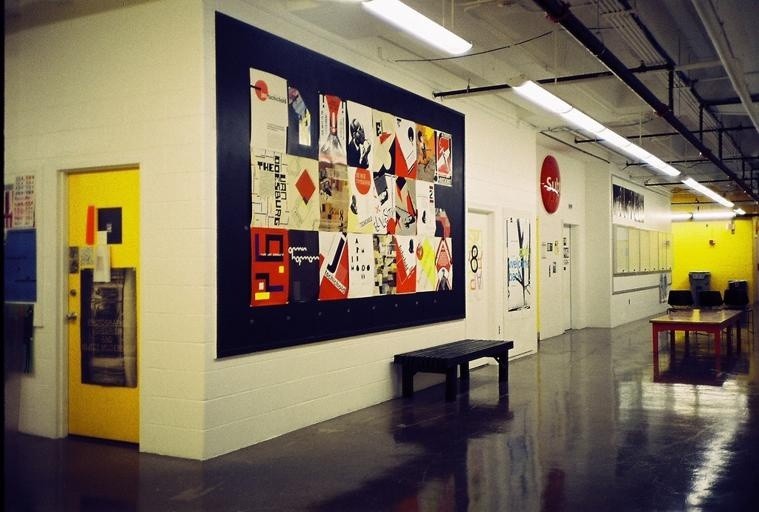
347;119;372;169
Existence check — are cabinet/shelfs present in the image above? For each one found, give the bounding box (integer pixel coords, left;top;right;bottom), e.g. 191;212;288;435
612;224;673;275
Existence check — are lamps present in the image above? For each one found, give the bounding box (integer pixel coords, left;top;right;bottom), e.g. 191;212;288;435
683;86;738;208
734;208;748;216
504;1;681;179
364;0;473;60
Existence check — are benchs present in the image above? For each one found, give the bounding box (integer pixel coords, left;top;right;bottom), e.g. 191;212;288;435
394;339;513;410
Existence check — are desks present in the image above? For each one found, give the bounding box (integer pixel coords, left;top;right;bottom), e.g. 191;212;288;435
649;309;743;383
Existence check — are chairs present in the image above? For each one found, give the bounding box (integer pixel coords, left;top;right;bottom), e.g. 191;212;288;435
668;285;750;308
